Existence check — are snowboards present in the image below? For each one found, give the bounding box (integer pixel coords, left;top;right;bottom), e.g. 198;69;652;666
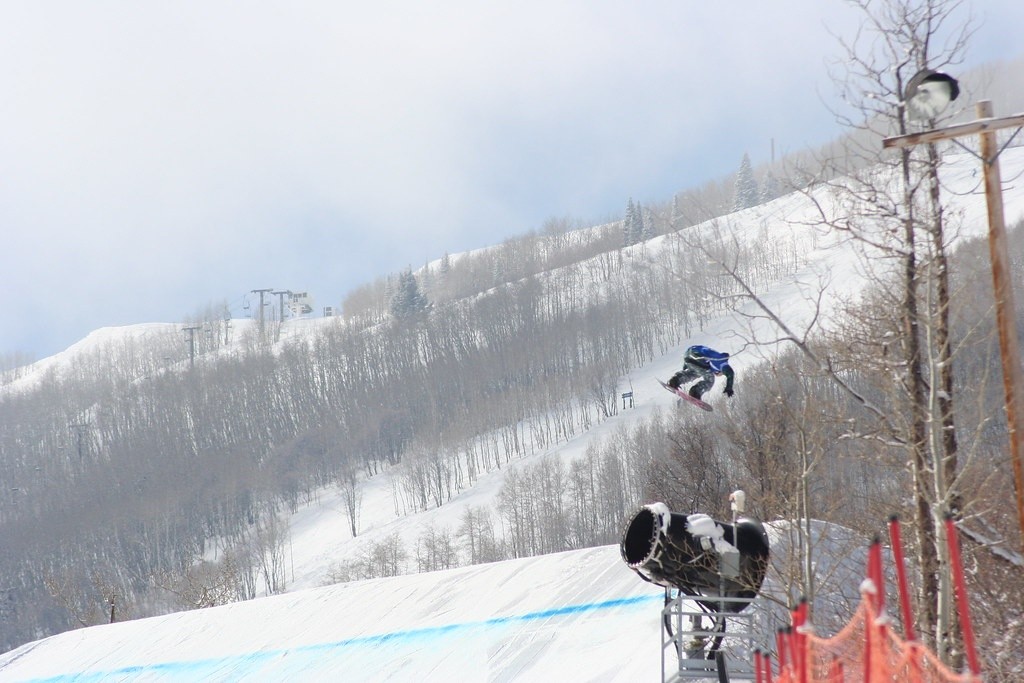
658;380;713;412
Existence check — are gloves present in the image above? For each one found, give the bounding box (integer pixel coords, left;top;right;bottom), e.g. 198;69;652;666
724;386;735;397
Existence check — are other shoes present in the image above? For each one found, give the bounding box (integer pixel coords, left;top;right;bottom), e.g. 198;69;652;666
690;386;702;400
668;378;683;393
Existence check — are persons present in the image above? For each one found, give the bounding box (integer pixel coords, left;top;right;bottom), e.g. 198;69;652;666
668;346;734;400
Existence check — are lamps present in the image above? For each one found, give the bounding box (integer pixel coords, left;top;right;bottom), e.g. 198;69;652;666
904;68;960;123
